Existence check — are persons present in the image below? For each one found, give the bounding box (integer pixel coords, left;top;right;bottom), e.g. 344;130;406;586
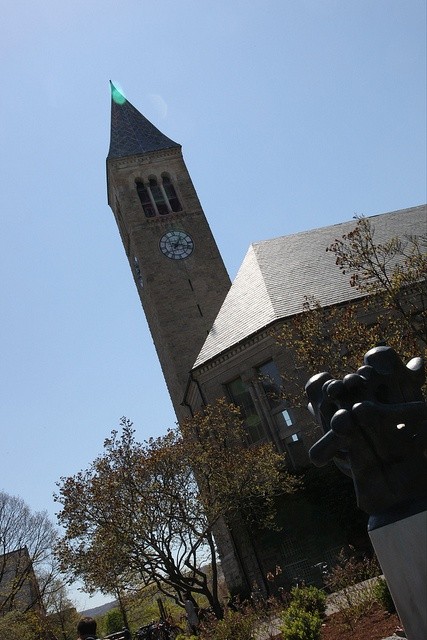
180;593;200;637
75;613;103;640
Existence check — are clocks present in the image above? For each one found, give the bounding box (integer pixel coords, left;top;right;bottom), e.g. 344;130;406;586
159;230;194;260
